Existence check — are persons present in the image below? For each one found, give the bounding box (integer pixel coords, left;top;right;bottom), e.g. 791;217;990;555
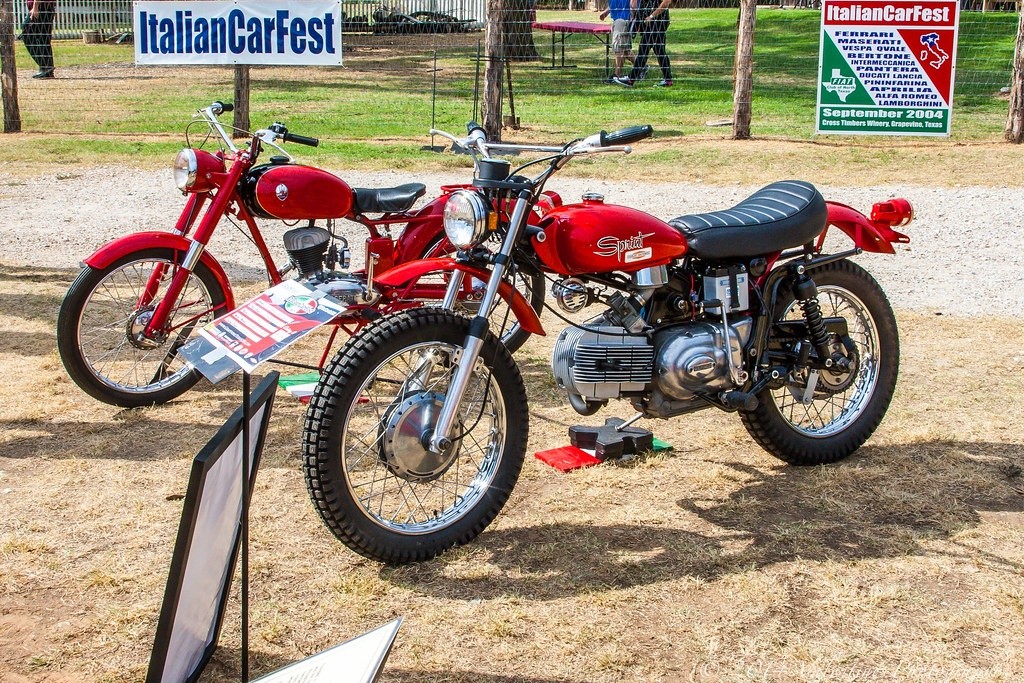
599;0;674;89
17;0;57;80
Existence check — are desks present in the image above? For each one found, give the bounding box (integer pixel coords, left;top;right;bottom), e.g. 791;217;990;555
532;22;614;77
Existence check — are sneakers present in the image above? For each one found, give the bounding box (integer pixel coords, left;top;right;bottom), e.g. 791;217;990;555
653;80;672;87
613;75;633;88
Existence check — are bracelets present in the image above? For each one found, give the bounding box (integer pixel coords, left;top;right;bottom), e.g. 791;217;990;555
650;14;654;19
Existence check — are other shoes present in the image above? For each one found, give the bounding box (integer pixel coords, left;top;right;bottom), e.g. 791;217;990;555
604;73;618;85
33;70;54;80
637;65;650;81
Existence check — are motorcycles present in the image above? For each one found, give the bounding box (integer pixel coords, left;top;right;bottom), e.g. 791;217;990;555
302;120;914;566
56;100;561;408
